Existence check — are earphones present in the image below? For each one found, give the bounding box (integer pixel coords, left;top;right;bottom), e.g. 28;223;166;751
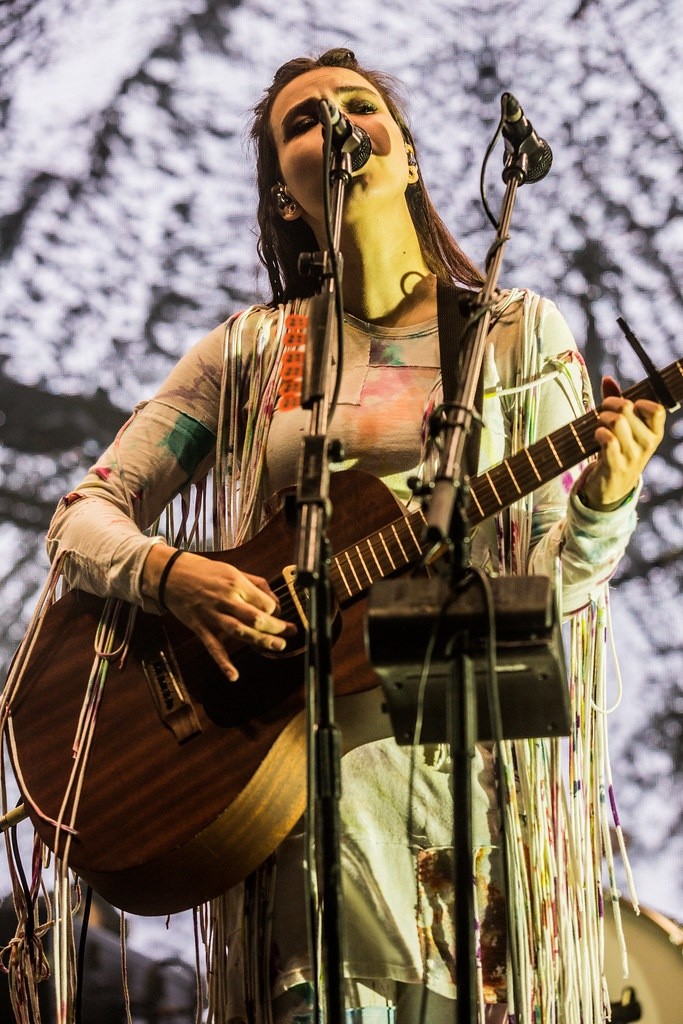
405;150;418;168
273;182;291;210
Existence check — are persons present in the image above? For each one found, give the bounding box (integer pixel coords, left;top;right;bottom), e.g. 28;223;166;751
45;51;672;1024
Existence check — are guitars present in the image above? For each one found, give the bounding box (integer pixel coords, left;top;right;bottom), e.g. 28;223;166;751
2;359;683;919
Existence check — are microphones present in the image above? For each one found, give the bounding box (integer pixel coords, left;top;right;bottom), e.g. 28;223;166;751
500;90;554;186
316;97;373;173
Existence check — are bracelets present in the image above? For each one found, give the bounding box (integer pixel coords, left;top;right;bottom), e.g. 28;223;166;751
157;549;186;608
